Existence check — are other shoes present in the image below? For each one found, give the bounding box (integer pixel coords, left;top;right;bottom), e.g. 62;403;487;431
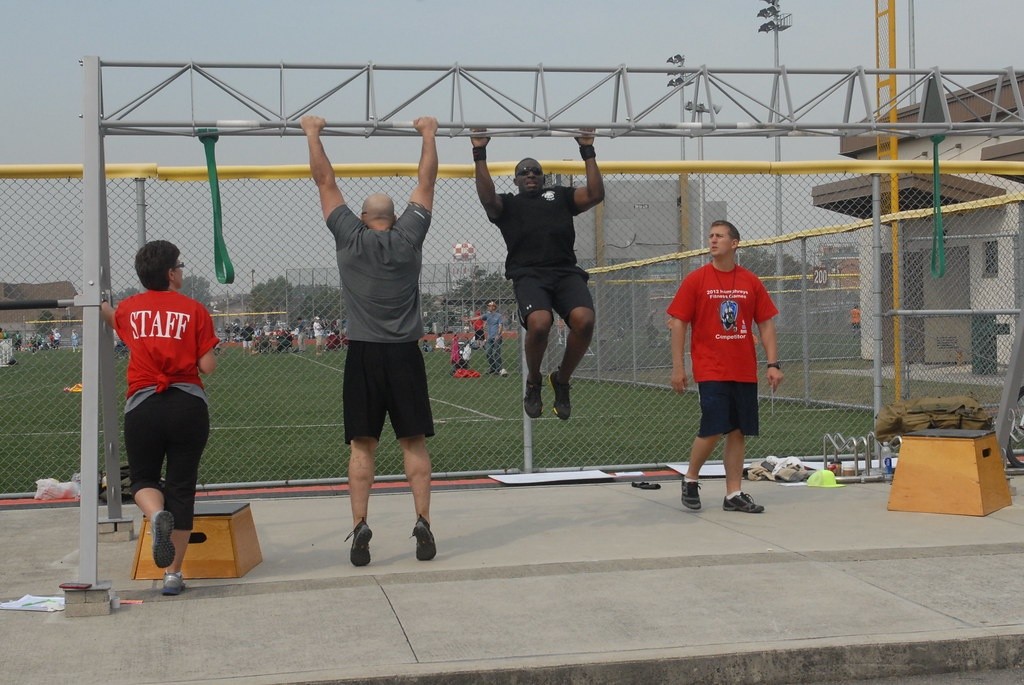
486;369;500;375
344;516;372;567
409;513;436;560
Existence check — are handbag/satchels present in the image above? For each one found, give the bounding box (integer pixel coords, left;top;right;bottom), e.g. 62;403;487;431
33;456;167;504
874;395;993;442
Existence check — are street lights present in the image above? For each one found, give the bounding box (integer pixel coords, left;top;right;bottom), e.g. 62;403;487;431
756;0;793;327
666;53;694;159
685;98;722;270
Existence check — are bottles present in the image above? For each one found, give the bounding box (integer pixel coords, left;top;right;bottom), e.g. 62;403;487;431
74;469;81;502
881;442;892;475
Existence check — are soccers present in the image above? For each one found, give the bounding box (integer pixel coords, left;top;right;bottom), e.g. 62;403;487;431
500;368;508;376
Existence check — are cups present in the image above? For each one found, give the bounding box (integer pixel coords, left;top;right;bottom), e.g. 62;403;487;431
833;461;842;476
111;597;121;609
843;469;854;476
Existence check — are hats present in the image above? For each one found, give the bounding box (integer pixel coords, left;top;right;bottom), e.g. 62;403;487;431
313;315;320;320
807;469;845;488
487;301;496;307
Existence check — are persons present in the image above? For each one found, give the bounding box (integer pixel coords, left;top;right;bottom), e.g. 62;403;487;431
101;240;220;591
70;329;81;353
666;220;784;514
0;327;61;355
313;316;348;355
423;333;445;352
462;300;504;376
300;115;439;570
224;317;306;358
470;127;606;419
453;336;471;368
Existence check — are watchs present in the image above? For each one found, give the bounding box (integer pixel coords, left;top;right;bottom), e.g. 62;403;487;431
768;362;781;369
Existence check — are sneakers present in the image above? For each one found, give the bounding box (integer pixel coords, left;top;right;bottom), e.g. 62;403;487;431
549;371;572;421
149;509;176;569
524;371;547;419
681;475;703;509
723;492;764;514
162;572;186;595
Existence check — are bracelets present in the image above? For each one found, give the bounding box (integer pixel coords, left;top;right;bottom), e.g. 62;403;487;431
579;145;596;161
473;147;487;161
497;331;501;336
101;298;108;303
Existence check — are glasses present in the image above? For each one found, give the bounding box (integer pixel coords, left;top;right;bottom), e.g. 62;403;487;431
171;261;184;272
516;167;543;180
